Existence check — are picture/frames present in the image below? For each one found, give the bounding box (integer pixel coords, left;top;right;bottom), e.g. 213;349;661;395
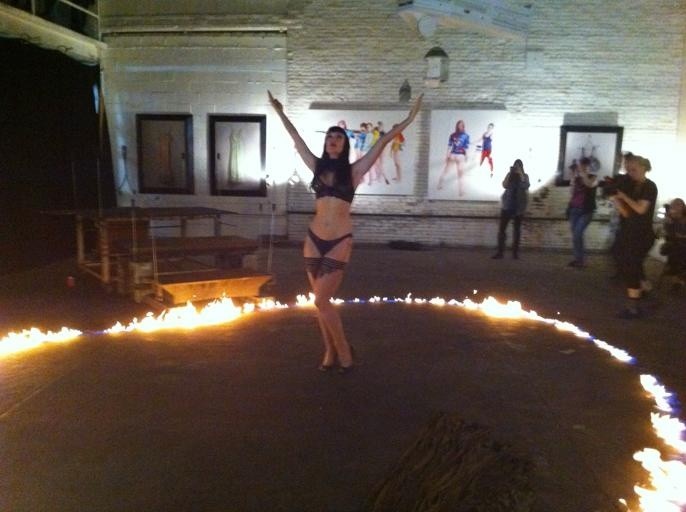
206;112;267;197
555;125;623;186
134;112;195;195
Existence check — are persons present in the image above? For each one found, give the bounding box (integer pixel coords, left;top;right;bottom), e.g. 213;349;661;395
264;85;428;378
472;121;497;182
489;159;532;265
566;153;601;270
437;117;469;199
315;119;405;188
662;198;686;283
608;151;654;319
602;149;633;291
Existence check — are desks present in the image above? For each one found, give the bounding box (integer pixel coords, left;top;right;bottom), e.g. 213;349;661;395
76;206;238;296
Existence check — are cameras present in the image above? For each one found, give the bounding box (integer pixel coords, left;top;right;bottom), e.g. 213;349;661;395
567;164;578;171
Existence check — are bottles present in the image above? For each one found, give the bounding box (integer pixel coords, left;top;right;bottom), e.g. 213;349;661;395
67;276;74;288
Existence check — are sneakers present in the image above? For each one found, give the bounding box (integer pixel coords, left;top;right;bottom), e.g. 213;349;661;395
490;252;520;260
569;257;585;268
608;292;658;318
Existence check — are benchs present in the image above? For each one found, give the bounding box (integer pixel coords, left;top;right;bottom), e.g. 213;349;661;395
114;236;263;262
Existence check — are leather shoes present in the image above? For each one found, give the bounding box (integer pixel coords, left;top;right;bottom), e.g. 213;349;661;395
319;353;336;372
339;346;355;374
